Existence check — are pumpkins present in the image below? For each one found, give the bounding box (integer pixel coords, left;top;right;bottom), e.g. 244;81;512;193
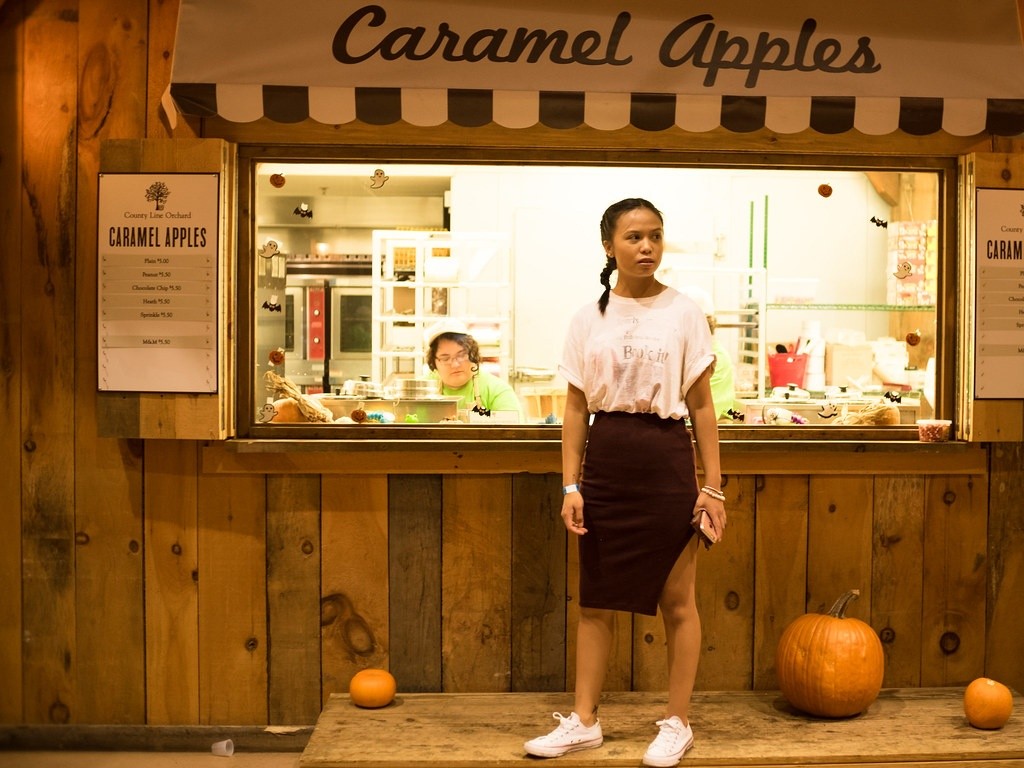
350;669;396;707
775;588;884;718
964;678;1012;729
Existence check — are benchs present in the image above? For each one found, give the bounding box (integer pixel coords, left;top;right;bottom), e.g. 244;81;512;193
294;686;1024;768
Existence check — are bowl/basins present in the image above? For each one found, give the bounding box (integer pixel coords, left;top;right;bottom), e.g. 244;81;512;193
915;419;951;442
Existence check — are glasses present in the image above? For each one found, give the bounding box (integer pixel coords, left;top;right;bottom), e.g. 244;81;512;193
434;353;470;363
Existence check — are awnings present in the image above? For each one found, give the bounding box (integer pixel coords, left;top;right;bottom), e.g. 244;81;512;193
156;0;1024;138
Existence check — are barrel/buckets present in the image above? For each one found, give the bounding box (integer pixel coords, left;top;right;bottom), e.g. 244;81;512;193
768;352;811;388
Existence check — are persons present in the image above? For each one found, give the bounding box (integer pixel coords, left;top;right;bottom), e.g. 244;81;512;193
523;198;728;767
422;320;524;422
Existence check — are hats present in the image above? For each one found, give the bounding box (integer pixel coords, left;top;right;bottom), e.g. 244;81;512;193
425;319;469;345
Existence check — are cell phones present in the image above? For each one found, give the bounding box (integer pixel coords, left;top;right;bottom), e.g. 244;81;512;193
700;510;717;543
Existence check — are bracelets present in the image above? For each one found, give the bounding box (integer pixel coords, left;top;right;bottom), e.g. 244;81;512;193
701;486;725;501
562;484;579;495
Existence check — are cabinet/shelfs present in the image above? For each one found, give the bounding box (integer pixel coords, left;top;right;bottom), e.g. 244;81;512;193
371;230;516;384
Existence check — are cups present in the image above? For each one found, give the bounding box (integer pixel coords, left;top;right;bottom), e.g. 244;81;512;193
211;738;234;757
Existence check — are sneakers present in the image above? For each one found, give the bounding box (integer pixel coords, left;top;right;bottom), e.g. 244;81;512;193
524;711;603;758
642;716;694;767
